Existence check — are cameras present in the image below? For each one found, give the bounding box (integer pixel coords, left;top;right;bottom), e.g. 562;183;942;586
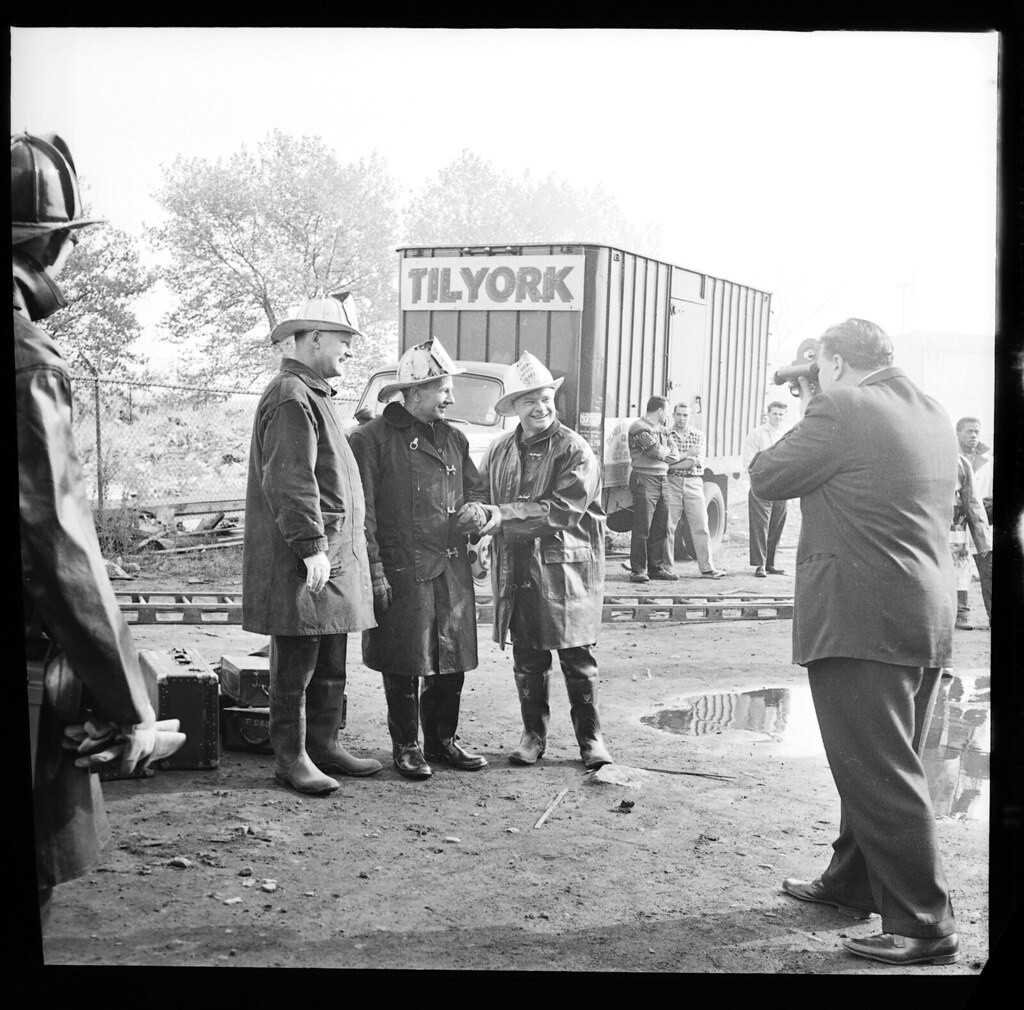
776;363;821;394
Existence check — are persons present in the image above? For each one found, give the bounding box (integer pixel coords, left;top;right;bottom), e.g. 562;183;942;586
480;349;614;768
628;394;681;581
742;401;789;577
749;318;959;965
242;298;383;792
349;335;490;778
11;129;158;922
950;416;990;629
668;403;727;577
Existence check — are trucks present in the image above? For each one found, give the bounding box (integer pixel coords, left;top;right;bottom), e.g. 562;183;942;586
348;242;773;606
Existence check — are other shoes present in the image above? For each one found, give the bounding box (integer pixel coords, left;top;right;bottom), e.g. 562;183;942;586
755;566;767;577
702;569;726;578
650;567;680;580
630;571;650;581
766;565;785;574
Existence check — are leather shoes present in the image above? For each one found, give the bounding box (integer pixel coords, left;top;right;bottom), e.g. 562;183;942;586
783;874;872;918
843;932;959;965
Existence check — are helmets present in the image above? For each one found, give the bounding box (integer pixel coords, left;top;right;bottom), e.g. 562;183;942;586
12;131;108;244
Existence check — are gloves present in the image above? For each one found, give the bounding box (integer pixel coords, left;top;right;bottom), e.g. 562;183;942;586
370;562;393;614
457;501;490;536
62;719;187;767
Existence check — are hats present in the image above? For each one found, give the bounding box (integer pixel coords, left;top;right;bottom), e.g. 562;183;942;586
270;292;363;342
378;336;468;402
495;350;565;417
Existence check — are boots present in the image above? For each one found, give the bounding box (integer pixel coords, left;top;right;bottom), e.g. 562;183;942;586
568;686;613;767
268;696;340;794
956;590;975;630
383;673;432;778
306;688;383;776
421;675;488;770
508;685;551;764
